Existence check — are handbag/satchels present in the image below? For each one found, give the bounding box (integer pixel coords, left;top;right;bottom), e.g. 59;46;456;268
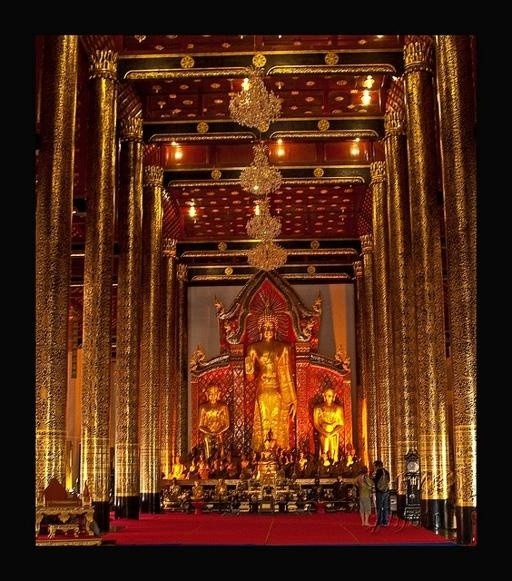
377;475;388;491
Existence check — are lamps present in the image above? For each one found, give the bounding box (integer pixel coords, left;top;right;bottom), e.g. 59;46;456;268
246;196;282;240
240;133;282;195
248;240;288;271
231;36;280;131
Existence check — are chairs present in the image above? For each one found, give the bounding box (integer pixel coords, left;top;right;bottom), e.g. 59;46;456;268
47;499;81;538
260;486;276;512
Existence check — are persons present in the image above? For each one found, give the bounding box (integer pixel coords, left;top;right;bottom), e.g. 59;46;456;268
314;387;344;466
245;314;297;450
199;385;229;479
163;432;360;503
357;461;390;526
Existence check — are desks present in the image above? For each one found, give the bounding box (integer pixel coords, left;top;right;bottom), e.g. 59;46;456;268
36;511;95;537
